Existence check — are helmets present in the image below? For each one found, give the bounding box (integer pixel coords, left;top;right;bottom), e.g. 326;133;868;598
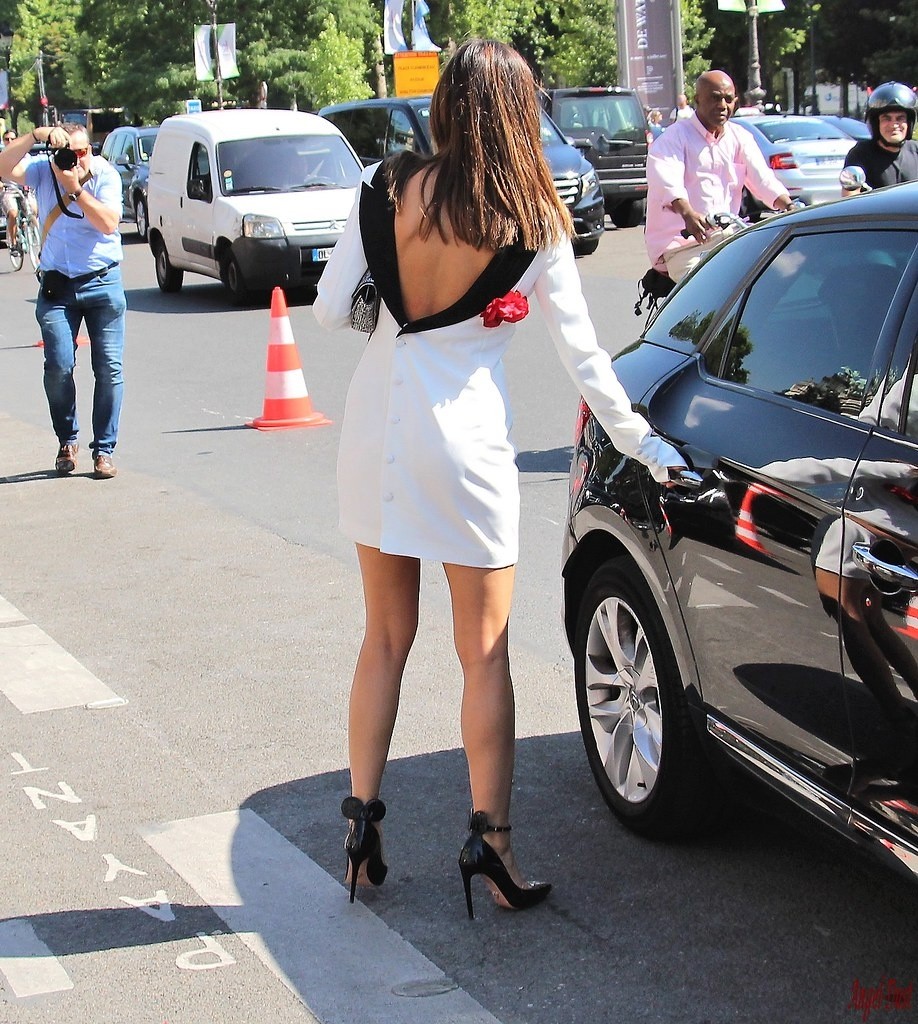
863;80;917;146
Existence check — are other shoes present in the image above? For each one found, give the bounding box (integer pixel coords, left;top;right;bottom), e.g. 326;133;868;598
11;245;20;257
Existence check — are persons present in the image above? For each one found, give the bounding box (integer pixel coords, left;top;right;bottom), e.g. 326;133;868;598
313;40;689;920
646;70;795;284
643;105;663;127
0;130;37;257
760;457;918;733
670;95;693;124
841;81;918;197
0;122;126;478
734;95;760;115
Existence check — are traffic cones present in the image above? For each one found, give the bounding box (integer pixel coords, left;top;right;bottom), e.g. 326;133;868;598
734;483;769;554
243;287;335;431
890;593;918;641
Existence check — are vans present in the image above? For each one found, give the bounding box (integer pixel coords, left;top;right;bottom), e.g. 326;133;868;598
144;109;366;306
532;85;654;229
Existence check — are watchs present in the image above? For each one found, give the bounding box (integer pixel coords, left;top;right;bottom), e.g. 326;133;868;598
69;187;83;201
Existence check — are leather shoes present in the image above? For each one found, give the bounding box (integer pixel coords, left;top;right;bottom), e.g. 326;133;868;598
94;456;118;480
55;443;78;472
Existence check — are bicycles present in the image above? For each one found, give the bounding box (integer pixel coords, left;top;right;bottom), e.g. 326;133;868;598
0;183;42;273
633;193;807;317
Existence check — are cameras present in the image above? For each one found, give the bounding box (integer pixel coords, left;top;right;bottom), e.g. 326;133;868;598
49;142;78;171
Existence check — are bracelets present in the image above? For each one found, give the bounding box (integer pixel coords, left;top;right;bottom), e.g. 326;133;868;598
33;133;38;141
786;203;794;211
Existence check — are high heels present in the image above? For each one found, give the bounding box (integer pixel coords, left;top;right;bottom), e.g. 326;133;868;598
458;810;552;922
340;798;389;905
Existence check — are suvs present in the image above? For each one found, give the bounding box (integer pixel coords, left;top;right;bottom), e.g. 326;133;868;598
313;94;605;256
100;125;163;242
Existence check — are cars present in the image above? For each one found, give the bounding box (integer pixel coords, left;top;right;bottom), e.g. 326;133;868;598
726;107;874;224
561;178;918;884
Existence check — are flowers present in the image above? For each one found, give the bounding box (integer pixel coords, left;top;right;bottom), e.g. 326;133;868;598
480;291;529;328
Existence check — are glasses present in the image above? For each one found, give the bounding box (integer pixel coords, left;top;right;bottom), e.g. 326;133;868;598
5;138;15;141
72;144;91;158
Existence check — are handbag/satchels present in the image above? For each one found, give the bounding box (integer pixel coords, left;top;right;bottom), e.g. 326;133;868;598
40;269;68;300
350;268;382;335
